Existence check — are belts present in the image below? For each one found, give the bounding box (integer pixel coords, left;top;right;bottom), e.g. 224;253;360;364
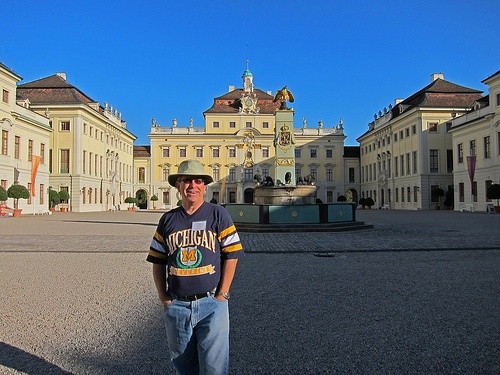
173;291;212;302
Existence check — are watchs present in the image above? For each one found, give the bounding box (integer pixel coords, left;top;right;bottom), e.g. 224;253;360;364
218;292;231;299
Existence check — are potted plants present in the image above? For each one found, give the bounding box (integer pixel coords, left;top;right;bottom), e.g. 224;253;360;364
58;191;70;212
150;195;158;210
49;190;59;212
125;197;138;211
6;185;30;217
487;184;500;214
359;197;374;210
433;188;445;210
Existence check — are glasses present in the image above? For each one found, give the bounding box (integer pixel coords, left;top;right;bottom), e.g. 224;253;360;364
176;177;206;184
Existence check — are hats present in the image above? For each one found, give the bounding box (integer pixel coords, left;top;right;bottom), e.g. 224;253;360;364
168;160;214;185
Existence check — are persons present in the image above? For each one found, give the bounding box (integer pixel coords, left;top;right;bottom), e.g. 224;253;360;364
145;160;242;375
